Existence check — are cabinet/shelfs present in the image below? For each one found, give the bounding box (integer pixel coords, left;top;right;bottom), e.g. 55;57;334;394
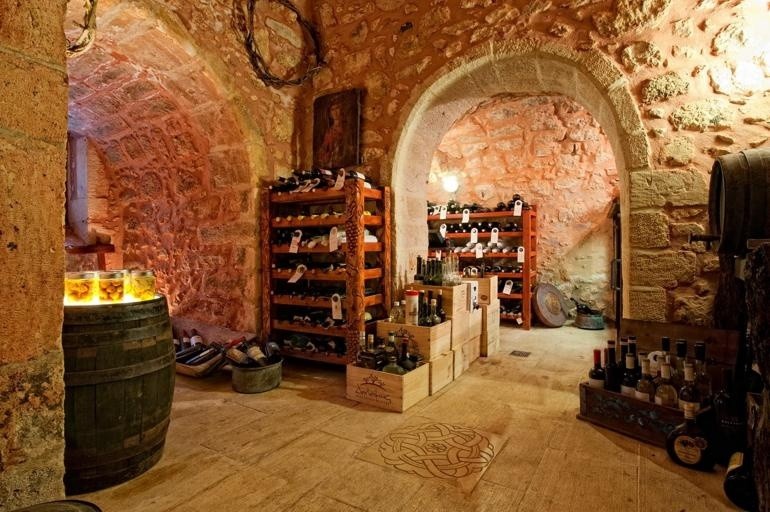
258;185;390;364
426;204;538;330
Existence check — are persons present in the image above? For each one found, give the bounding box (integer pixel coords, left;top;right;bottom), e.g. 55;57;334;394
319;96;354;169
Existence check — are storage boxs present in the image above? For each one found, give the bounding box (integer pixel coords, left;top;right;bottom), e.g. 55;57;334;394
346;275;500;414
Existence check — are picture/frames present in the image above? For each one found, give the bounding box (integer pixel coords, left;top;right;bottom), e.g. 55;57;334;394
313;88;361;169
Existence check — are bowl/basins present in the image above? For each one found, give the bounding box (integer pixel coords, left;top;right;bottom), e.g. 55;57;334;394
231;361;284;395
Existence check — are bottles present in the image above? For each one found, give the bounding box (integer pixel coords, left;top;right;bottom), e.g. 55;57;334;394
269;165;384;355
414;253;462;286
568;297;591;314
427;195;533;321
588;335;710;416
666;405;718;472
711;370;743;453
734;323;758;396
359;332;416;373
722;445;759;512
212;338;279;365
170;326;246;363
390;289;446;326
